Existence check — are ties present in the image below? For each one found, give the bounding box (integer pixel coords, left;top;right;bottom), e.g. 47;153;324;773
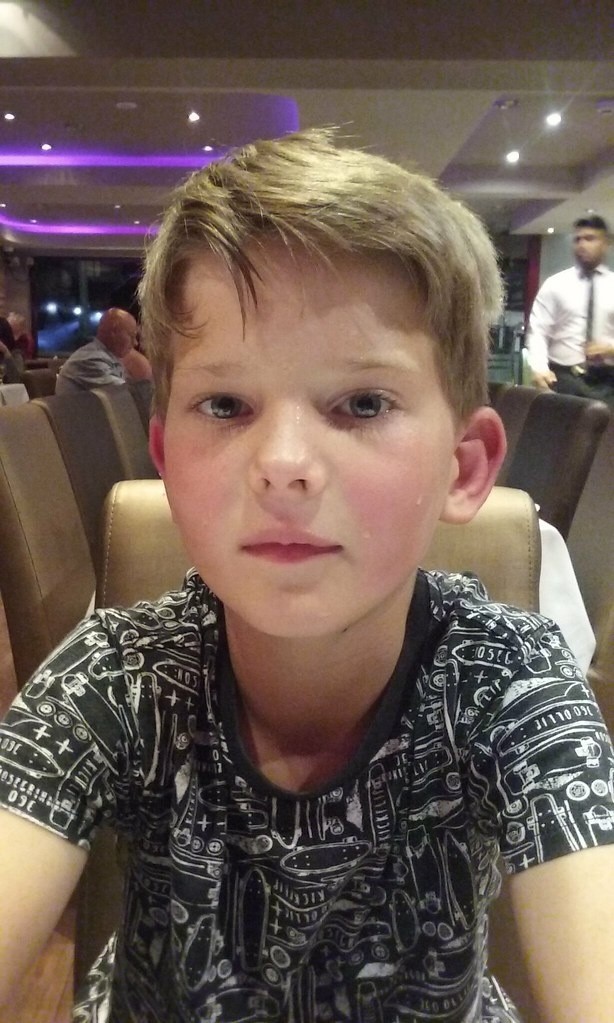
584;273;594;347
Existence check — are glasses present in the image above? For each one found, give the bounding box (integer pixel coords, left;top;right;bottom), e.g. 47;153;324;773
572;234;597;243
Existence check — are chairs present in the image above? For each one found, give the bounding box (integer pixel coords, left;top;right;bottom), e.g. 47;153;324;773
0;349;614;1022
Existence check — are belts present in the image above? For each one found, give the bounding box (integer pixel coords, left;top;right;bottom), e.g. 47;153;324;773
547;362;614;378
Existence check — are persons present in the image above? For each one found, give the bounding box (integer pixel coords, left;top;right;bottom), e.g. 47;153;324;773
0;315;14;362
54;307;136;397
4;123;614;1023
525;217;612;403
8;311;34;367
